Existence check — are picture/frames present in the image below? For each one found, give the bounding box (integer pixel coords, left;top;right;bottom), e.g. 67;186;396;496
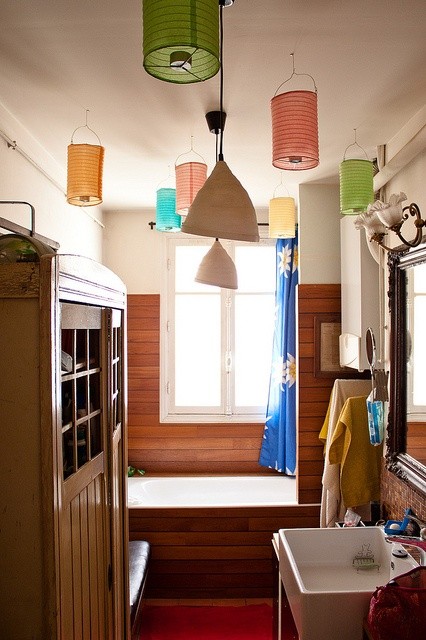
313;315;366;379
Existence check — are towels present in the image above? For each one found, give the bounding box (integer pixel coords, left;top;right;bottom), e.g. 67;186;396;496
318;385;333;457
328;394;380;507
319;379;372;528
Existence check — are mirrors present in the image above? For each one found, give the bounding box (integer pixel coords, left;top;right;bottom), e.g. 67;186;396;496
383;235;426;500
365;327;375;367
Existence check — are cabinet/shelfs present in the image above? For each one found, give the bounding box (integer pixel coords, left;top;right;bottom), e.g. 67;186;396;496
0;253;135;640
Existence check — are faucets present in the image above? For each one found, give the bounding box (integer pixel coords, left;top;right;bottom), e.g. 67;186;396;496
385;515;426;566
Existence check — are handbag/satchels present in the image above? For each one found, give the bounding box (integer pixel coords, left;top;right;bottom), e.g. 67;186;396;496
367;566;426;640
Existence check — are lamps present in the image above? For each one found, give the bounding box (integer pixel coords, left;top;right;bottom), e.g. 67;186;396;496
270;54;320;171
366;191;426;248
353;206;419;252
174;136;208;216
268;169;297;239
193;238;238;290
154;165;181;233
141;0;219;85
178;0;261;243
66;110;105;207
339;129;374;215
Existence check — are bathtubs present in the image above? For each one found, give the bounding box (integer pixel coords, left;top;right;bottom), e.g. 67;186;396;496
127;476;297;506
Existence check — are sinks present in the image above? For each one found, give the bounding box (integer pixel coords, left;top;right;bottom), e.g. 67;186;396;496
279;526;419;594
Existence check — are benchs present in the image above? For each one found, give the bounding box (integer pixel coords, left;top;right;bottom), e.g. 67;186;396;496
129;540;152;633
